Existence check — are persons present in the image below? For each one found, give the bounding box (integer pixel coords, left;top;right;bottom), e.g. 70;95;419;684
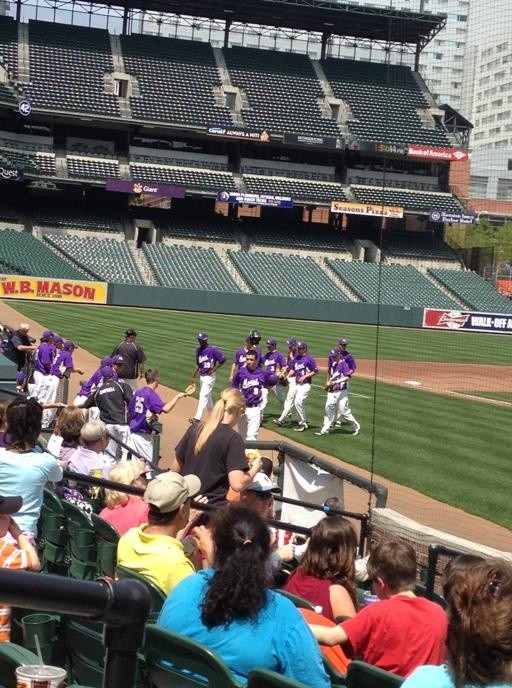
4;324;85;429
398;556;512;688
74;329;187;479
189;331;226;424
1;389;449;688
228;328;360;442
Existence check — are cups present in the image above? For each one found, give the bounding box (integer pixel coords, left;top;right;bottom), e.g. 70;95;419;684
14;662;68;687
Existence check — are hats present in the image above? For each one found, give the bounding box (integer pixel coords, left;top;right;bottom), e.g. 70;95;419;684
195;329;348;357
143;471;202;513
43;329;138;378
0;496;23;514
246;471;283;495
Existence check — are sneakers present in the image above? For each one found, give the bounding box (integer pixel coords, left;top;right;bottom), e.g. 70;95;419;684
273;411;360;436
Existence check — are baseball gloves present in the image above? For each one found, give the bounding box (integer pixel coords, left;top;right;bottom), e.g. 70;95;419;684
186;383;196;396
